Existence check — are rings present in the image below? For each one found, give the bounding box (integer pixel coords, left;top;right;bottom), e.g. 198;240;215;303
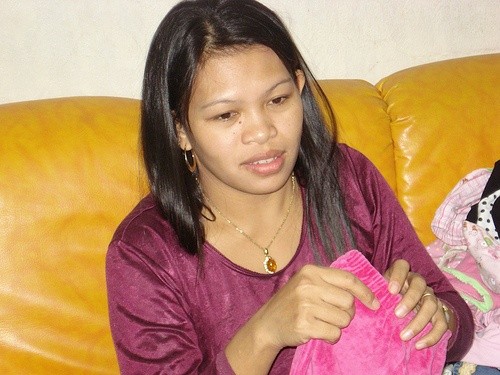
422;291;439;309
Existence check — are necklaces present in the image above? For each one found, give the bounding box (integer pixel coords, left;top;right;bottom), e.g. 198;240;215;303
197;173;297;275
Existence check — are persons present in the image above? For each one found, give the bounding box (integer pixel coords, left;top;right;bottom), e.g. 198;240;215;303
106;0;474;375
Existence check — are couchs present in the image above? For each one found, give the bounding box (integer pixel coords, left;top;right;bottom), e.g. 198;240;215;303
0;53;500;375
